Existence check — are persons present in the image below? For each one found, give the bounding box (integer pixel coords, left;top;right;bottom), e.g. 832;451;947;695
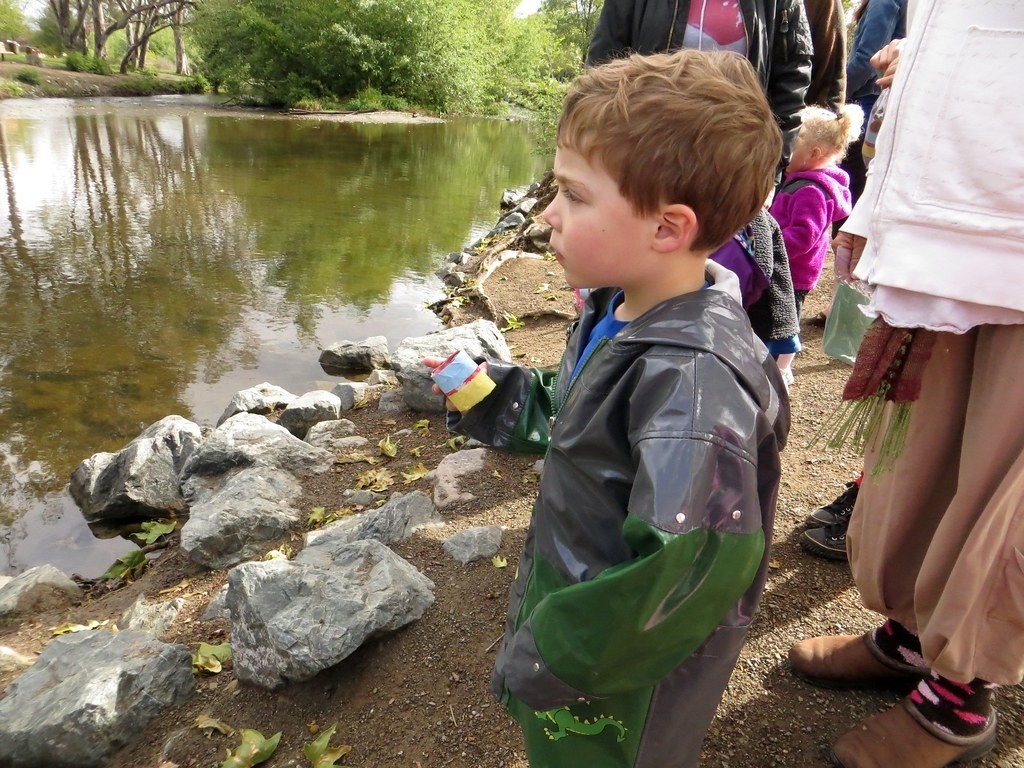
576;208;802;398
424;48;793;768
583;1;813;215
791;1;1024;768
767;104;863;325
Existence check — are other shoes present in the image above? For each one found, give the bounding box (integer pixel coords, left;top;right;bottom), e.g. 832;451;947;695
830;696;996;768
789;628;929;692
799;525;851;561
808;483;860;528
803;311;826;326
